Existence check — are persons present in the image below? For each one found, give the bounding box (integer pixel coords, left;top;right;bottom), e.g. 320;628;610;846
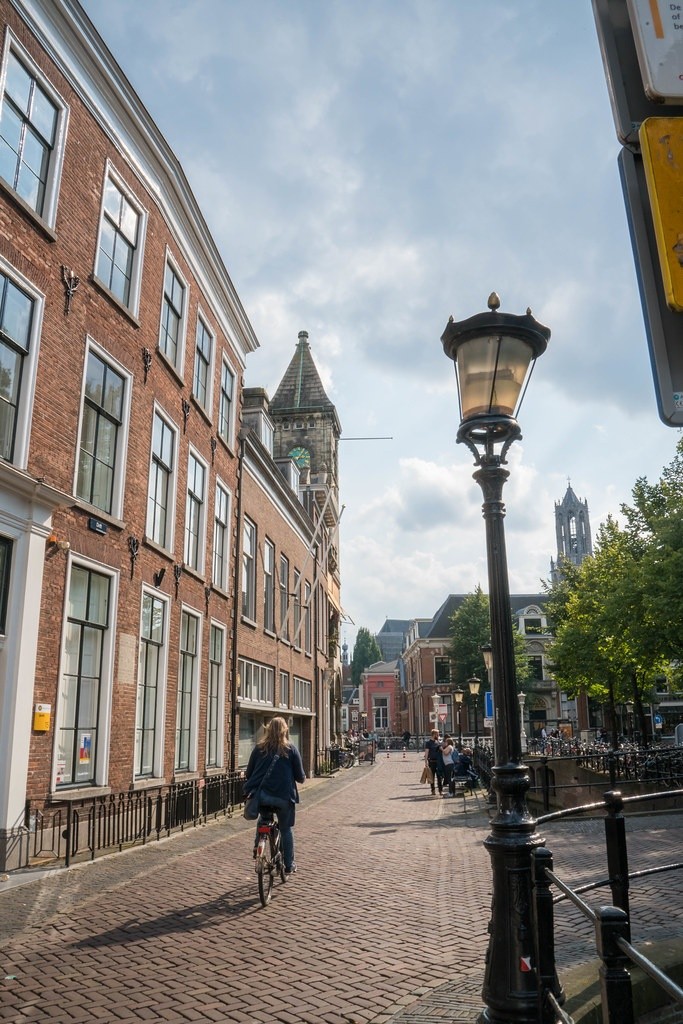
425;729;479;798
245;717;306;874
346;728;377;755
541;727;568;756
596;727;608;742
401;729;411;748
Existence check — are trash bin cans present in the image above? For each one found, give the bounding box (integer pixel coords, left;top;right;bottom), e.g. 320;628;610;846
331;749;340;769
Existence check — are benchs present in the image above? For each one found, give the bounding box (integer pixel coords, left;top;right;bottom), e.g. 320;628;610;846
452;773;472;797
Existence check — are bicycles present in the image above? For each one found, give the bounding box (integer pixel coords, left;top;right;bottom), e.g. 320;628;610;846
376;729;416;751
239;773;307;910
337;741;356;769
528;732;683;790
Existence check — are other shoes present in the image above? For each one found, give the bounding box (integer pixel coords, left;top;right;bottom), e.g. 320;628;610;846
432;791;436;795
443;793;453;798
440;791;444;795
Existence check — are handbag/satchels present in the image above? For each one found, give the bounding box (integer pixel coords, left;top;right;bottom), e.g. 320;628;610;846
420;766;434;784
244;794;258;820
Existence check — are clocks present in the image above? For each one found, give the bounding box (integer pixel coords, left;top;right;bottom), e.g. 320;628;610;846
286;446;313;469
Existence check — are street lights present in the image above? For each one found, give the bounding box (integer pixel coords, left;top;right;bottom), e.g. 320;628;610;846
466;674;485;774
450;684;464;755
438;292;572;1024
517;688;529;754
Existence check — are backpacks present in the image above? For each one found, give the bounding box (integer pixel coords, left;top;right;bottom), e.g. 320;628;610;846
450;748;459;765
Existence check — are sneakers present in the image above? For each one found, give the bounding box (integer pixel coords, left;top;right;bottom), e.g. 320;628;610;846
253;848;257;859
285;861;297;874
